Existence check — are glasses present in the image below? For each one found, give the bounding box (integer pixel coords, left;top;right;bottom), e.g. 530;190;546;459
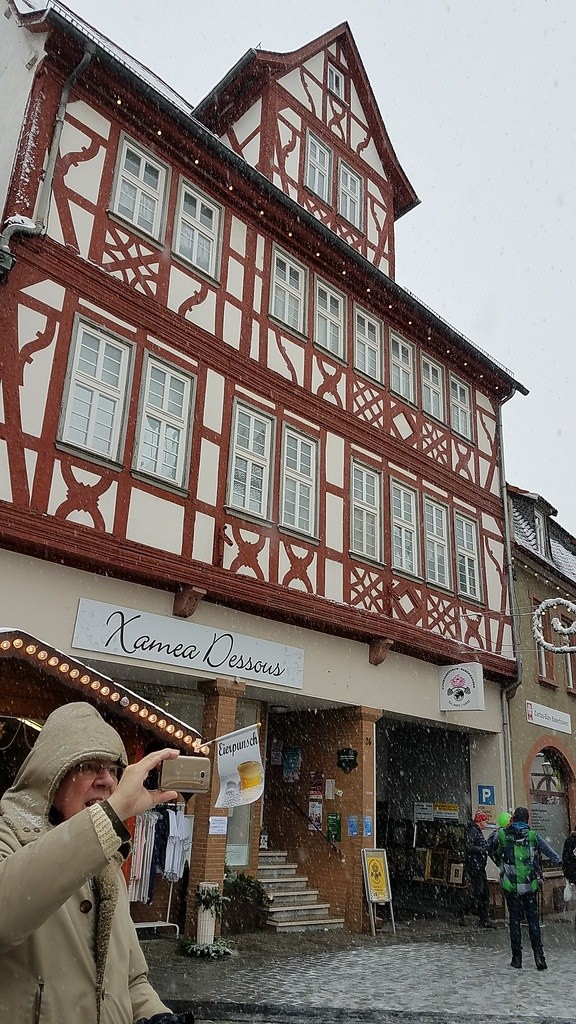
69;762;124;777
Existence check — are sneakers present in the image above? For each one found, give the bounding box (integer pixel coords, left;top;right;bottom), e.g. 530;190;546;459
455;908;469;926
479;914;497;928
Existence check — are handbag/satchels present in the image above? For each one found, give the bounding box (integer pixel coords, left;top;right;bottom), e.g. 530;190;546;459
564;877;576;902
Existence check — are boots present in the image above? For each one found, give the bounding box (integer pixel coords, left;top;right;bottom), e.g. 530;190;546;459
534;948;547;971
511;950;522;969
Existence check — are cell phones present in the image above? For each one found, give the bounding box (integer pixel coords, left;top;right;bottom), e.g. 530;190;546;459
160;756;211;793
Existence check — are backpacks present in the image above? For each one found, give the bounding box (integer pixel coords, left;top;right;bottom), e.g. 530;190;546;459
498;828;538;896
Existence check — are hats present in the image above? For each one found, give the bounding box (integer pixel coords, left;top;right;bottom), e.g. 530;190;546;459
474;810;489;823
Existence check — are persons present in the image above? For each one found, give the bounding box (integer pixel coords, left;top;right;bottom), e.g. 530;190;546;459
562;826;576;887
487;807;565;971
309;813;320;824
456;809;497;928
0;702;194;1024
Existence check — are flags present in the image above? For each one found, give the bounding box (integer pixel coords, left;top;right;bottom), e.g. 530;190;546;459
214;724;265;808
